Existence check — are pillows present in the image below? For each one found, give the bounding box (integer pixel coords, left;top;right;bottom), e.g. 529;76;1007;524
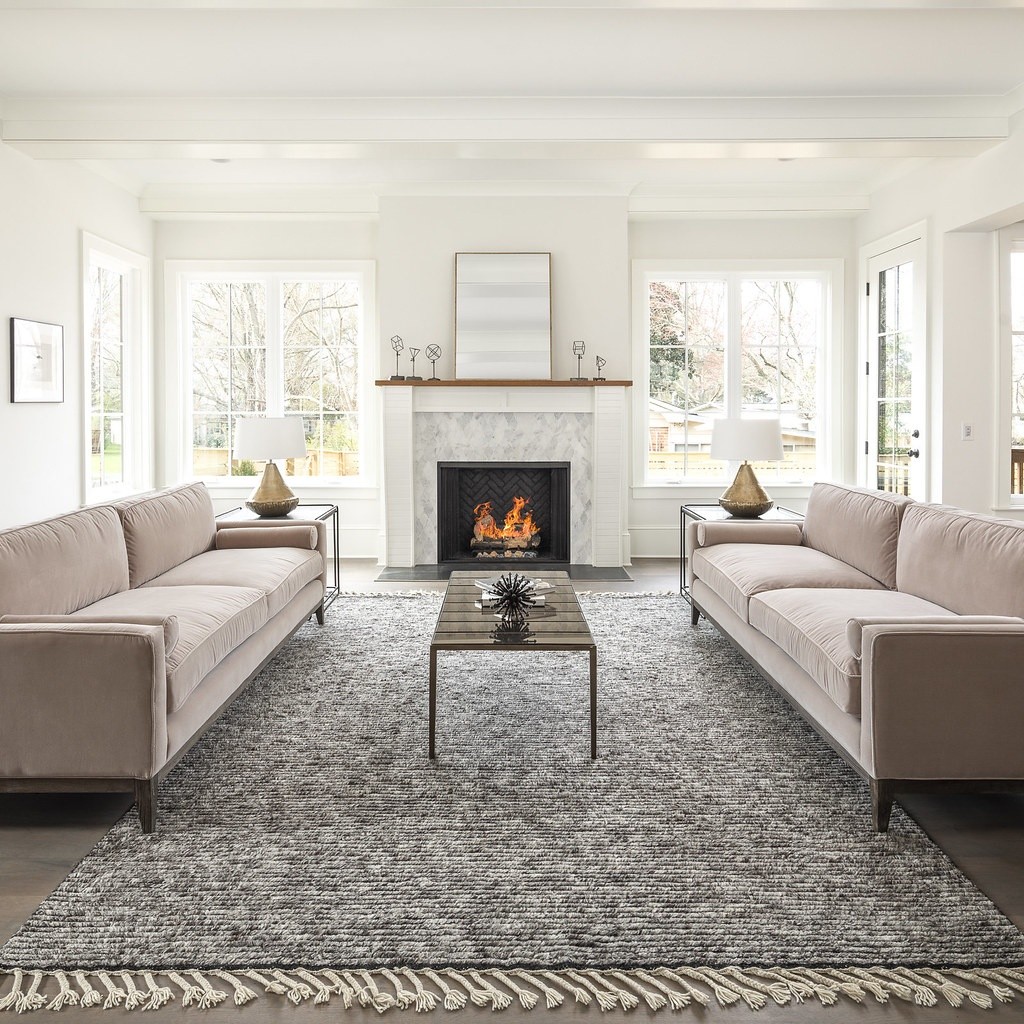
0;613;179;659
216;525;319;550
845;615;1024;661
697;522;803;548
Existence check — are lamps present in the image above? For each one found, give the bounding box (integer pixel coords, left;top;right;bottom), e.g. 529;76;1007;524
710;417;784;517
232;417;307;517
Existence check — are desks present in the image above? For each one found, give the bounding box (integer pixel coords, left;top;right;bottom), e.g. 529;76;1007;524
679;503;805;619
429;570;597;759
214;503;340;621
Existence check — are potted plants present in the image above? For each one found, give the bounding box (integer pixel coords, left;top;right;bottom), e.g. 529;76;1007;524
197;418;360;476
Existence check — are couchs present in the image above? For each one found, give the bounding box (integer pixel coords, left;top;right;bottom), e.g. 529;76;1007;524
0;481;327;834
686;481;1024;833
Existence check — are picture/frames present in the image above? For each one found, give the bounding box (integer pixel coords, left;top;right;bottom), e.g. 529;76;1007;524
9;317;65;404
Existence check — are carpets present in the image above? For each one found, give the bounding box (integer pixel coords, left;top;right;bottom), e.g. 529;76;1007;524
0;590;1024;1013
